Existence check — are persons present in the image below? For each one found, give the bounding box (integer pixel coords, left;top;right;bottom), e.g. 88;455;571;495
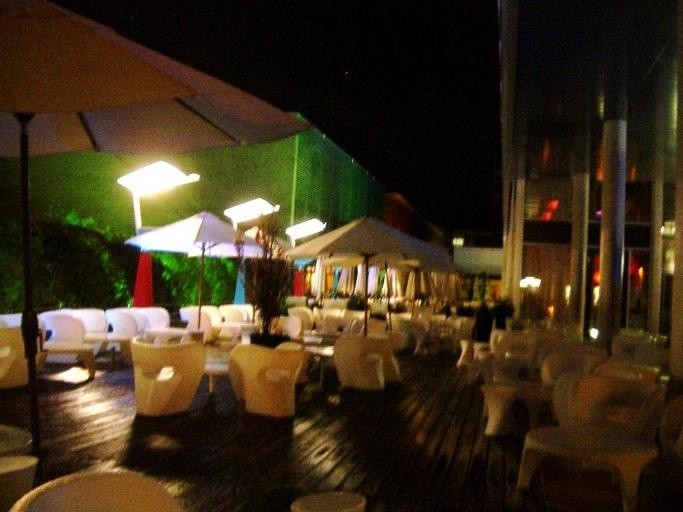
637;398;683;512
471;279;506;360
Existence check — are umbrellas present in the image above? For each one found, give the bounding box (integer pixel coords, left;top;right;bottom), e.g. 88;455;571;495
0;0;315;456
284;215;449;336
124;211;264;329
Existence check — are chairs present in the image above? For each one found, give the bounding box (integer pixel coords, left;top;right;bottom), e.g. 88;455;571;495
0;295;683;512
